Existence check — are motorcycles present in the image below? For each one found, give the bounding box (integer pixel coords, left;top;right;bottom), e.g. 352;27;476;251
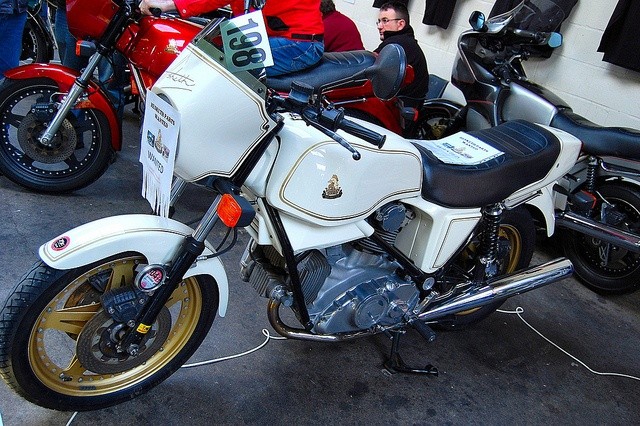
20;0;235;115
1;0;584;413
0;1;418;193
420;0;640;296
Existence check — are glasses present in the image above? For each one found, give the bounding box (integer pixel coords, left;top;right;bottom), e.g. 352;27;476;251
376;18;401;23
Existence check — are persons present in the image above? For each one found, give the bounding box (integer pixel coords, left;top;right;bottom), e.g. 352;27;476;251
138;0;325;77
0;0;27;76
320;0;365;51
372;0;429;108
54;0;87;72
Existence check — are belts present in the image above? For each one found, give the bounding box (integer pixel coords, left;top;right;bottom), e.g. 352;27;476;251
292;32;324;40
